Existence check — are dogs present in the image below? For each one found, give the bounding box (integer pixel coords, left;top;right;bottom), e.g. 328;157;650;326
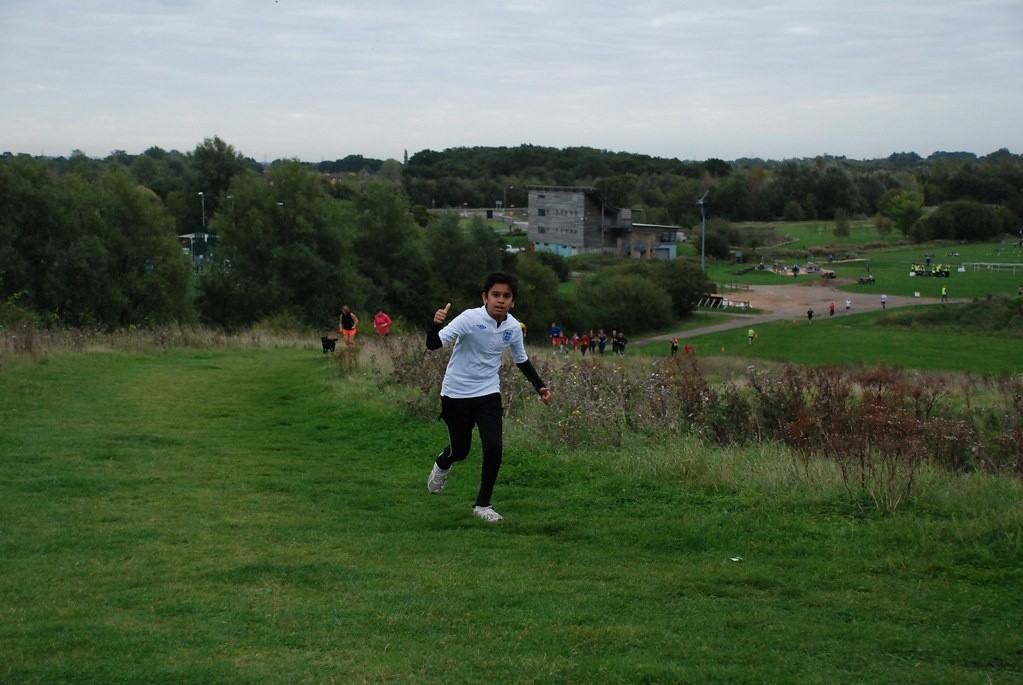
321;337;338;354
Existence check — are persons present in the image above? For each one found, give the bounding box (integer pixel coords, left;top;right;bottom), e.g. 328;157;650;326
339;306;357;347
735;228;1011;346
374;309;392;338
426;271;551;522
670;336;678;355
512;314;634;355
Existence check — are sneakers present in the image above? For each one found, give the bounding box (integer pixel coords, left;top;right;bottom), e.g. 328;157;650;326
428;453;452;494
473;504;503;522
